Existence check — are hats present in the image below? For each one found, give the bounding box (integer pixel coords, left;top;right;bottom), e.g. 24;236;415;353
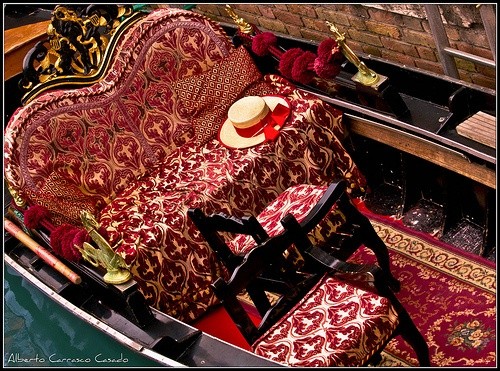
218;95;291;149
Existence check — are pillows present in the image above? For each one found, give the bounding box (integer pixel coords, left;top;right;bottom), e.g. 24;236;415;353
170;45;284;148
32;173;102;236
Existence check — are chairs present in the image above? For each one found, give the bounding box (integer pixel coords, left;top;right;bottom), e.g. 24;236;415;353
210;213;434;368
185;175;403;319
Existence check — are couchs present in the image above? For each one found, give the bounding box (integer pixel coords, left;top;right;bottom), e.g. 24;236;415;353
3;5;367;329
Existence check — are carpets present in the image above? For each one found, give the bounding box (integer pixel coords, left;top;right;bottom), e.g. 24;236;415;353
223;205;496;367
